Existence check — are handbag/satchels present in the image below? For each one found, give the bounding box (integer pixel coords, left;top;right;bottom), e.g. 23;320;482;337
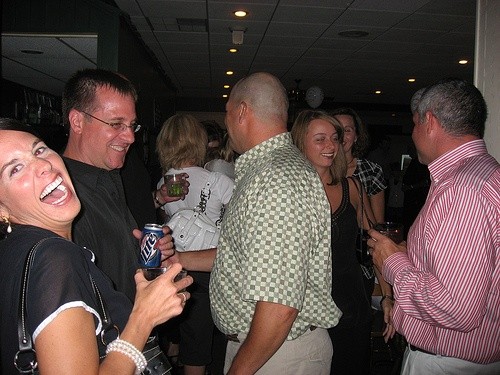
356;227;375;267
14;236;173;375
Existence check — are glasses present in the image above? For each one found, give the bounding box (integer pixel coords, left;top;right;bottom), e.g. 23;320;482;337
77;110;142;132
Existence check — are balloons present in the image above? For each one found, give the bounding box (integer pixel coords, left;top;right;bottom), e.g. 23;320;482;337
305;87;325;109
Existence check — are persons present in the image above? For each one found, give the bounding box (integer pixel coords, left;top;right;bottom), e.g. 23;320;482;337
200;121;241;180
332;109;388;225
61;68;176;305
291;110;396;375
367;79;500;375
153;113;236;375
367;136;431;242
0;118;193;375
119;141;190;232
161;72;343;375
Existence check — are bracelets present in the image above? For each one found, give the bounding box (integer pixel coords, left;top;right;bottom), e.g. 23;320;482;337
153;190;166;207
106;339;147;375
380;295;394;309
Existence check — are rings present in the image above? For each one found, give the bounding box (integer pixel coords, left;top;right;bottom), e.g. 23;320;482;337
389;324;391;325
177;292;187;303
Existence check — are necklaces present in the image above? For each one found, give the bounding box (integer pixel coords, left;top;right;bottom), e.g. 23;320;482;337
347;157;354;165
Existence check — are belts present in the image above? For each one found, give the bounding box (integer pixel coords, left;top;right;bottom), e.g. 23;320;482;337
406;339;436;356
225;325;316;343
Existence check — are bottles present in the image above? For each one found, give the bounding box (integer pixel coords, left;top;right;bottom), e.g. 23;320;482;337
26;102;38;128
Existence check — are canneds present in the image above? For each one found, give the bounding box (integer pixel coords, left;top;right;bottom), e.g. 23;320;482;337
139;224;164;268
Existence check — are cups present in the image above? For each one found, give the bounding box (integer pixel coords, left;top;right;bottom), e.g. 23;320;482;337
373;223;401;244
163;172;186;197
143;268;187;320
